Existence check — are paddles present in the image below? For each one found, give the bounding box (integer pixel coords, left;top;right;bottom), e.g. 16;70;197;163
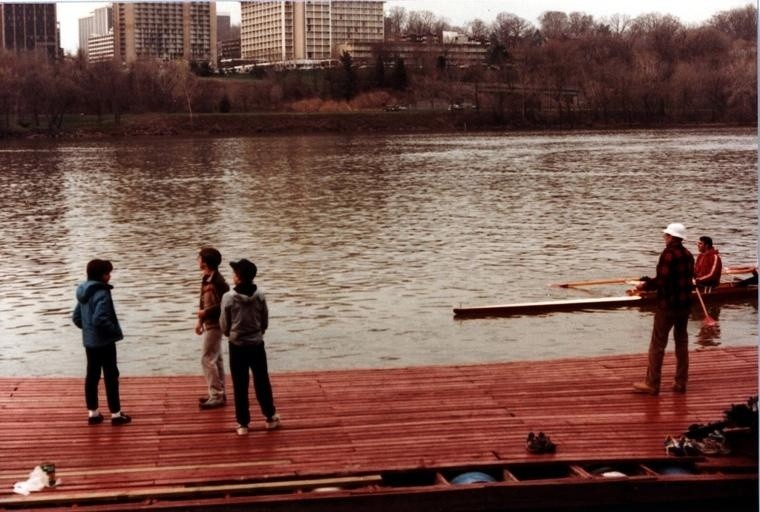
547;279;629;288
696;286;715;323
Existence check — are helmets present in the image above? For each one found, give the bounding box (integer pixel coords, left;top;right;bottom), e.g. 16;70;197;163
662;223;688;241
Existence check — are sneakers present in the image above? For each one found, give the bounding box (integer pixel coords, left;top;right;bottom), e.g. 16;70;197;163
199;395;225;409
265;414;280;428
235;423;246;436
111;415;132;426
89;413;104;424
634;383;657;394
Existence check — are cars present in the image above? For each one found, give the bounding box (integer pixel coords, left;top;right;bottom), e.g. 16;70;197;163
383;104;408;112
450;104;477;112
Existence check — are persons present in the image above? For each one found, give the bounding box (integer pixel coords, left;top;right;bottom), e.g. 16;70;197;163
692;236;723;290
632;221;695;395
72;258;132;426
219;257;280;436
193;246;230;411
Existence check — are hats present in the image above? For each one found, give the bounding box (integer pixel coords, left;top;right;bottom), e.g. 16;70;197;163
230;259;257;279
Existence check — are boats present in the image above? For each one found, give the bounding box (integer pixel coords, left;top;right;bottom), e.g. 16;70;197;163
453;272;760;315
